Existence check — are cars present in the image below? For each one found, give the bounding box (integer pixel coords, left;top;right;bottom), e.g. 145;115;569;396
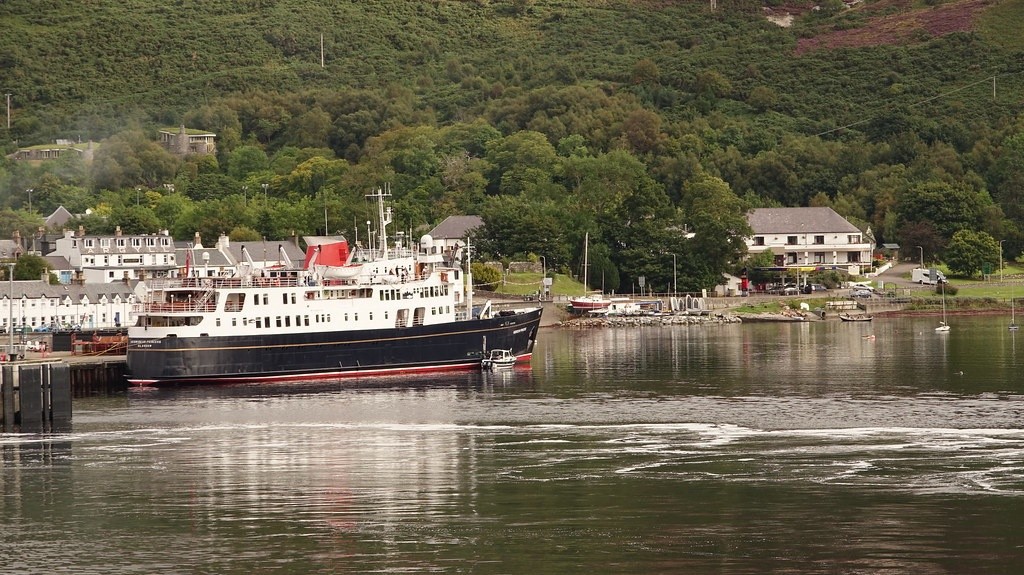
33;324;51;332
768;286;783;294
853;283;875;292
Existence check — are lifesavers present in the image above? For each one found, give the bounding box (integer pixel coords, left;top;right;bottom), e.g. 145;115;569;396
441;273;448;282
275;280;280;286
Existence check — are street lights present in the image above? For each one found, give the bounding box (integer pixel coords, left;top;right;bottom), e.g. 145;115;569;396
25;188;34;215
135;187;141;207
916;246;923;270
6;263;17;355
241;186;249;207
1000;240;1006;282
793;250;799;287
162;184;174;195
262;183;269;209
667;253;676;298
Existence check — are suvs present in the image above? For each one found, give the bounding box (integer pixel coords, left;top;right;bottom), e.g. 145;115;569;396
851;290;872;298
779;285;801;295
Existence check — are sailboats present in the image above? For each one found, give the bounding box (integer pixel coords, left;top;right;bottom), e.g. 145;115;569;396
935;281;950;331
1008;283;1018;329
570;233;611;309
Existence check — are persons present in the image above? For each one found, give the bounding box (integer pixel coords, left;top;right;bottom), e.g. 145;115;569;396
92;331;99;353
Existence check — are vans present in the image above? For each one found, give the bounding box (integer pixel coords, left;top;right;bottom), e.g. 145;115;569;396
812;284;827;291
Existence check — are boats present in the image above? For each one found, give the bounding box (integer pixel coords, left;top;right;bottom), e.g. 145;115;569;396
125;182;545;385
480;349;517;369
839;315;872;321
615;294;713;316
588;310;608;314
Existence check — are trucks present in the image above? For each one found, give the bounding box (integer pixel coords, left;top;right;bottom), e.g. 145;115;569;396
912;269;949;285
